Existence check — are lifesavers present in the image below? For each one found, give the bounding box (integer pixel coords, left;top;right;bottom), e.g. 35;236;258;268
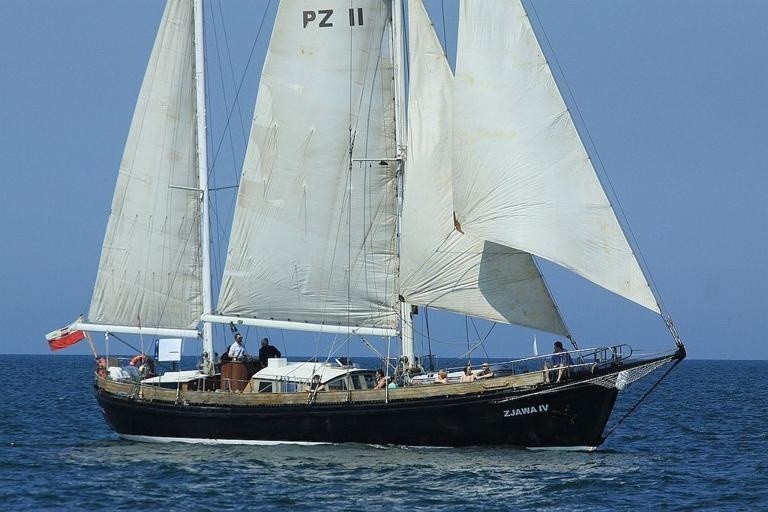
130;356;155;381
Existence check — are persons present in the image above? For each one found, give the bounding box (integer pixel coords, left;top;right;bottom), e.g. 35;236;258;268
374;369;387;388
305;375;325;391
220;345;232;360
460;364;478;382
543;341;575;385
433;369;449;383
227;332;246;360
475;362;495;379
258;338;281;368
388;374;400;389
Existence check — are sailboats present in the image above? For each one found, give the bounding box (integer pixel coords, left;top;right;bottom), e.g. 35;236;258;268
43;2;688;451
